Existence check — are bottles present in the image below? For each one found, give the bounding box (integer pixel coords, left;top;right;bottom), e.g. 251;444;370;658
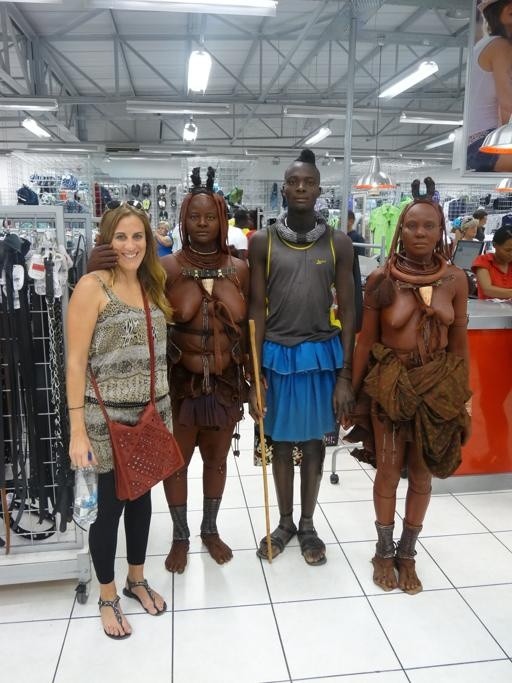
72;450;100;525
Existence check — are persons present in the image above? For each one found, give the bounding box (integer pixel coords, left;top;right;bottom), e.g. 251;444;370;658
234;210;250;237
471;208;488;241
64;200;167;639
342;177;471;594
459;215;478;242
153;220;174;258
346;210;366;256
87;166;251;573
246;209;257;236
247;148;355;565
470;224;511;299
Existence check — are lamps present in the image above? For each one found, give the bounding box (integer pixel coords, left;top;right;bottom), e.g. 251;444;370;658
305;125;457;151
283;103;382;121
184;14;214;96
126;97;233;117
373;41;442;103
21;113;53;138
181;115;201;145
398;105;464;130
0;94;60;112
83;1;280;18
494;177;512;193
352;33;399;191
478;113;512;154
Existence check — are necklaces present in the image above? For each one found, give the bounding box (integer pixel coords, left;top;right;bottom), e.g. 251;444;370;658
395;251;440;276
180;245;225;268
276;210;328;243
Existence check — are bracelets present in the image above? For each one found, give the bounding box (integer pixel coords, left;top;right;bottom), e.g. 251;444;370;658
66;405;86;410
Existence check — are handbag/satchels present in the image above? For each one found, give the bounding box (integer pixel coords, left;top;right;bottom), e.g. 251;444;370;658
106;402;185;501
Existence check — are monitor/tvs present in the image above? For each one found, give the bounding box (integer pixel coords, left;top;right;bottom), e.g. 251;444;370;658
451;240;486;271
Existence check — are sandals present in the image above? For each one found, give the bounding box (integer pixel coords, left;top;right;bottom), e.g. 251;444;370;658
396;556;423;594
123;577;167;616
98;595;132;640
256;524;298;559
371;553;398;591
297;526;326;566
